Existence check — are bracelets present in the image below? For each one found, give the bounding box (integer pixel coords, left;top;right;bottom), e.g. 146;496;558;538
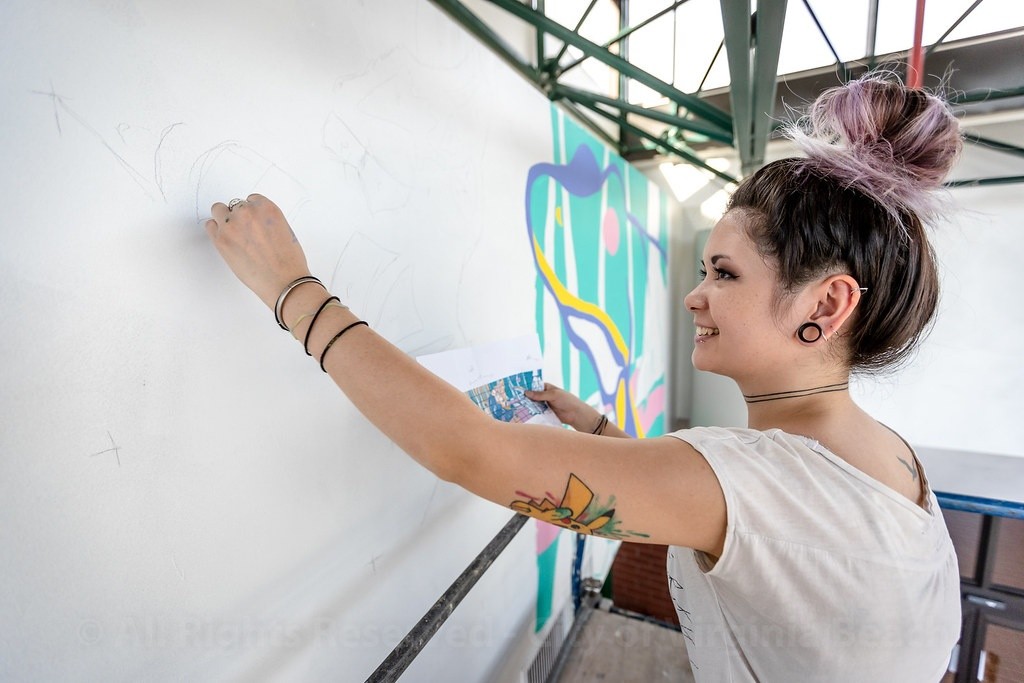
274;275;370;373
591;414;608;435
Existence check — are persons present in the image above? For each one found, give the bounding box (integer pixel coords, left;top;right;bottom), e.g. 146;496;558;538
204;70;963;683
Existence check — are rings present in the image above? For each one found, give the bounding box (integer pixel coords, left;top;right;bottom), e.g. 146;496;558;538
227;197;245;212
